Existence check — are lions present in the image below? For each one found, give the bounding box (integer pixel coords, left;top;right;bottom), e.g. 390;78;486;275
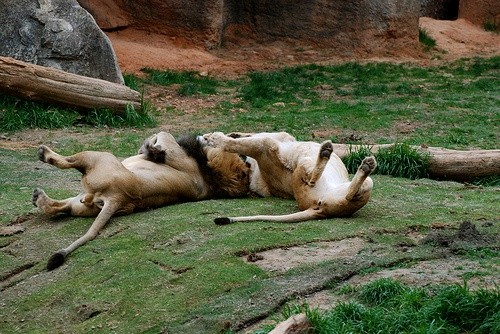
31;131;377;272
